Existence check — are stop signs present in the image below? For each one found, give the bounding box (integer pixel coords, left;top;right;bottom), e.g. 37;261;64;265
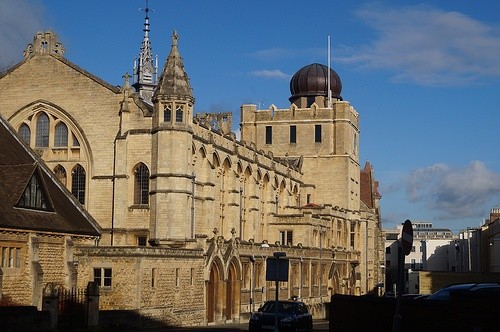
402;219;413;256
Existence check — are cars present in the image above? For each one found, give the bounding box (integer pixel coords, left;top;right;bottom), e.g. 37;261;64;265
396;282;500;304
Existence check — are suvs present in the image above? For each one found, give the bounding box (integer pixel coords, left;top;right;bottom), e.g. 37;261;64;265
249;299;312;332
